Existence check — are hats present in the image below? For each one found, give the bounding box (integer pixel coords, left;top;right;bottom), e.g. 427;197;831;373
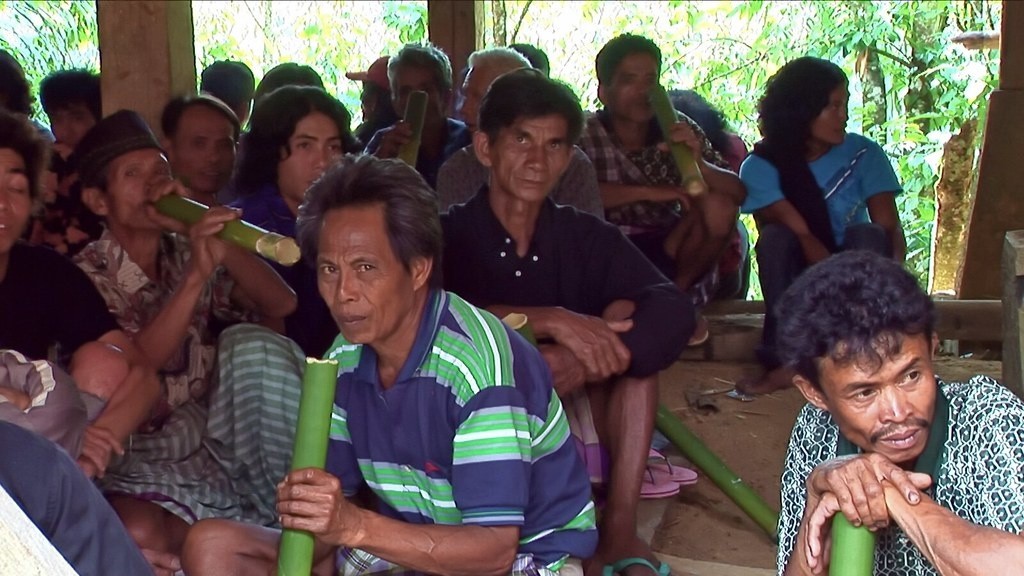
345;55;392;89
201;58;255;100
68;110;159;178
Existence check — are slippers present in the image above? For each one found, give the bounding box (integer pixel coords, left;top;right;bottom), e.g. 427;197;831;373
645;449;697;486
688;320;709;346
638;470;682;498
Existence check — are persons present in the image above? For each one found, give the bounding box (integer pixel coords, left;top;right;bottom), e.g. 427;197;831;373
0;33;752;576
737;57;908;397
777;251;1024;576
